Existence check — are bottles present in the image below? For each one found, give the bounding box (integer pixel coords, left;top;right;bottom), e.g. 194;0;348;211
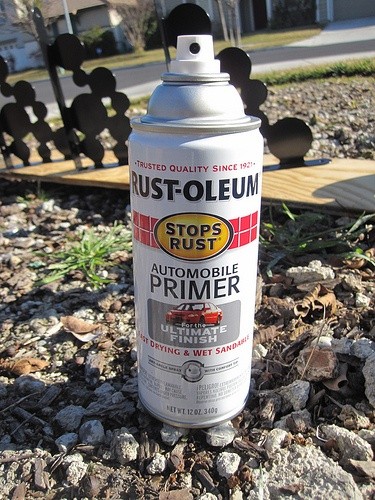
128;33;262;426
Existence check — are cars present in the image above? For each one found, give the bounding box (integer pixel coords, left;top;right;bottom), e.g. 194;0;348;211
166;302;223;328
